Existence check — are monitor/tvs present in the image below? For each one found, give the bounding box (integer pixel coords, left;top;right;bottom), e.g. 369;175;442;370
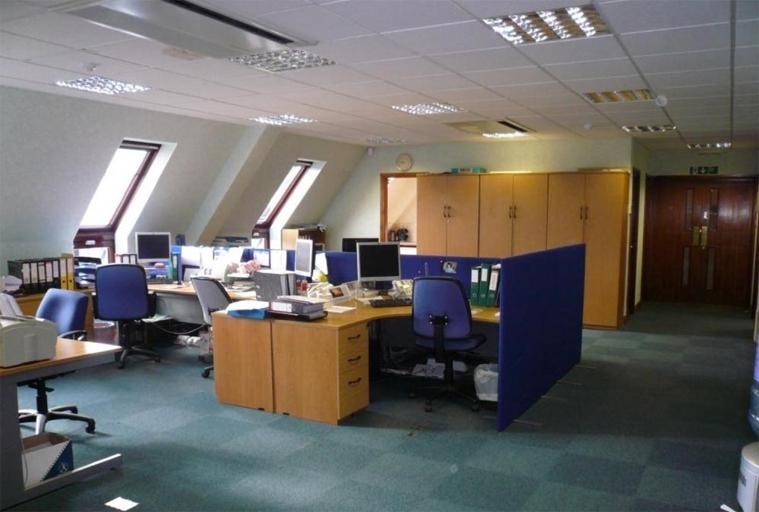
315;242;325;251
294;238;313;283
356;241;402;295
135;231;171;270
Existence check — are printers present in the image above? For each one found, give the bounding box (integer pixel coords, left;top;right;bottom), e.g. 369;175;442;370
0;293;57;367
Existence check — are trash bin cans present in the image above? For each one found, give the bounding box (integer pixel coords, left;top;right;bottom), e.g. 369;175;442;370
93;321;116;343
473;363;498;412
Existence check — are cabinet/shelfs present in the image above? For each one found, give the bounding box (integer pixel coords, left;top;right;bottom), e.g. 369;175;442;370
416;173;479;258
479;173;545;258
14;288;93;336
147;280;274;414
546;171;629;329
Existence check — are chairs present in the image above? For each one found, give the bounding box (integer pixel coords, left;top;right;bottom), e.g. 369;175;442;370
191;276;232;377
95;264;161;367
18;288;96;435
412;276;487;413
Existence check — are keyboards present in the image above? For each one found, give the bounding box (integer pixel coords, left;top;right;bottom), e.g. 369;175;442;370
147;277;169;284
368;298;412;307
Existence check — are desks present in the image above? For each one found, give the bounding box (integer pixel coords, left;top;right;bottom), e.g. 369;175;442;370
271;304;500;427
1;338;123;510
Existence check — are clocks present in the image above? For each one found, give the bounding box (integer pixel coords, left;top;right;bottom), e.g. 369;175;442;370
393;153;413;170
